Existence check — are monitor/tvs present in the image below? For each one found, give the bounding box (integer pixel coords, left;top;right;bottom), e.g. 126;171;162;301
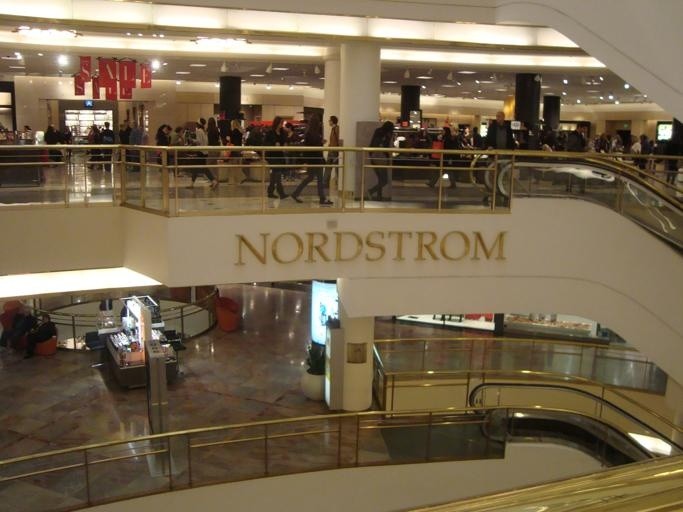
656;120;672;141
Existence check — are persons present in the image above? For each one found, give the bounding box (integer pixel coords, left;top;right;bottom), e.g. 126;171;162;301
22;314;56;359
17;110;682;207
1;305;37;355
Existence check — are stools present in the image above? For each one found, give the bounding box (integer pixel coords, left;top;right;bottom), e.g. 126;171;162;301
17;335;28;349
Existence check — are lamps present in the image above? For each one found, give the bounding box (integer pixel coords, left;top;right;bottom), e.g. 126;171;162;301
404;68;410;78
221;61;229;72
447;72;453;81
266;64;272;74
314;65;320;74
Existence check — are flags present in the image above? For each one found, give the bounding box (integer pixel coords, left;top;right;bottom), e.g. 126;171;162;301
74;56;153;102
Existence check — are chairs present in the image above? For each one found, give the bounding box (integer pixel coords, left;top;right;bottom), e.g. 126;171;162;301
34;328;58;355
215;297;241;332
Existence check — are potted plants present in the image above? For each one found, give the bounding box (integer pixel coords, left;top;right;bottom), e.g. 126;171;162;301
301;347;325;401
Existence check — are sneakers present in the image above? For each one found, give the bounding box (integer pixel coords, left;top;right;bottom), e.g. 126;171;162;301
0;346;7;352
8;348;16;354
364;189;371;201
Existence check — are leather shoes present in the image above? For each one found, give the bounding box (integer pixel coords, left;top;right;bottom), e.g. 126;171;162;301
23;353;33;359
291;194;303;203
446;184;456;188
186;177;247;191
320;199;332;204
426;182;434;188
27;343;35;353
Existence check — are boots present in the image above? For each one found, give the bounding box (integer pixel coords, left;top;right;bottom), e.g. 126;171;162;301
277;186;289;199
267;186;277;198
